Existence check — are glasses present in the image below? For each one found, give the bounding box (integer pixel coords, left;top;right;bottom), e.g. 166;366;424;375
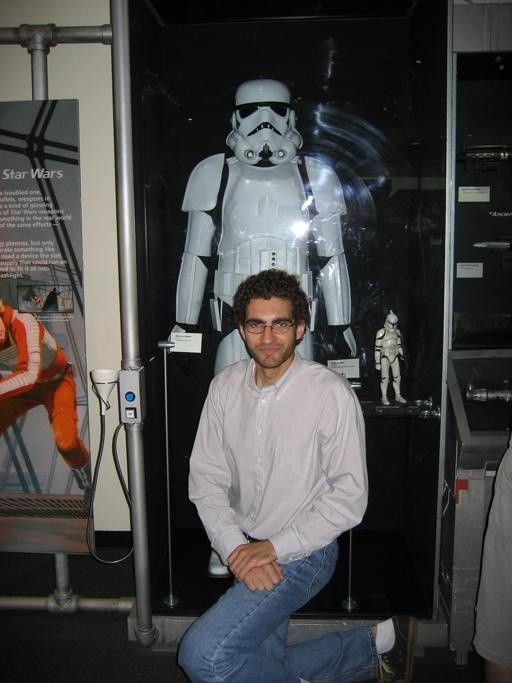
242;320;298;335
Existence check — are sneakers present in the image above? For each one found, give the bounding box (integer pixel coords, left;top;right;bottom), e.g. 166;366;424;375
379;615;417;682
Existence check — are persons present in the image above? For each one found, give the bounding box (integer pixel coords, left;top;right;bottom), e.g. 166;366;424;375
175;267;420;682
42;287;63;313
0;300;92;491
471;431;511;683
